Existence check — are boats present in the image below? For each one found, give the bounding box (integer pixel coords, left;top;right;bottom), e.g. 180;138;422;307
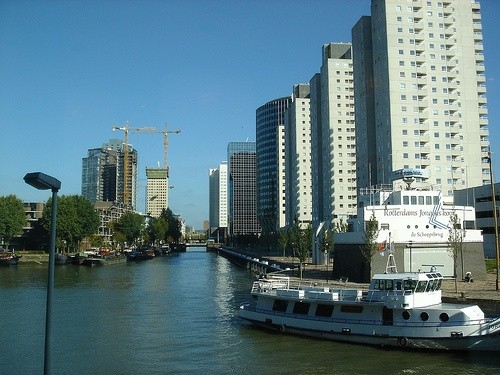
68;242;186;263
0;250;21;266
239;232;500;353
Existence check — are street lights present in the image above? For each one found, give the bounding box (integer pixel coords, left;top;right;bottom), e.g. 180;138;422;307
23;172;62;375
